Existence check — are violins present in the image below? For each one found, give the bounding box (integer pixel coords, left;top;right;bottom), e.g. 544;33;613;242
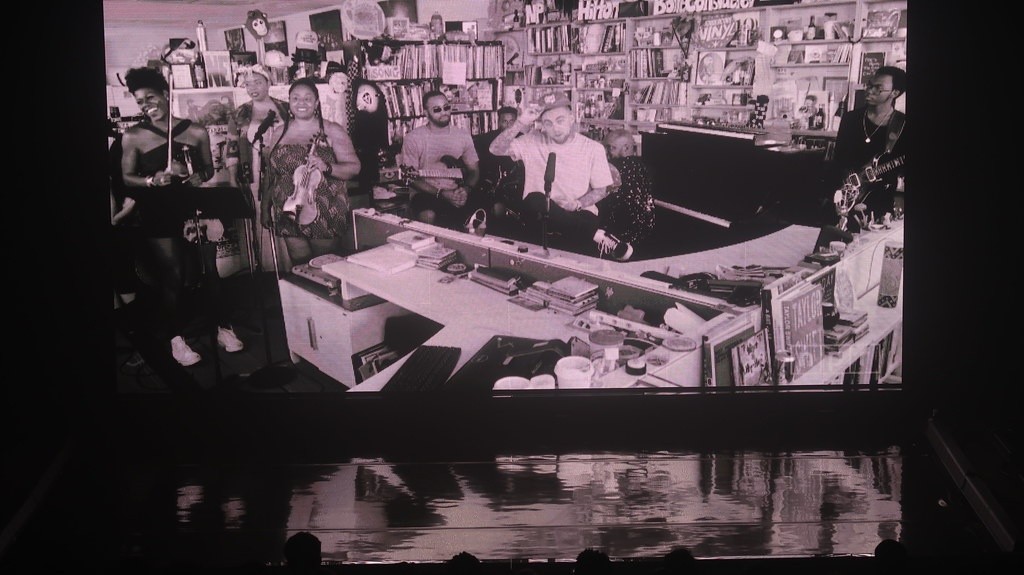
181;145;225;246
282;127;328;227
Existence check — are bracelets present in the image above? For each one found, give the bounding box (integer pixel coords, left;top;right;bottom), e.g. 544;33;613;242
145;176;156;187
199;170;209;181
323;161;332;179
436;188;442;200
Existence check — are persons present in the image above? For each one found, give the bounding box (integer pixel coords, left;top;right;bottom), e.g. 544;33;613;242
108;67;244;368
599;135;656;243
490;92;634;263
824;67;907;233
0;498;1024;575
471;107;525;219
401;91;480;229
225;64;361;276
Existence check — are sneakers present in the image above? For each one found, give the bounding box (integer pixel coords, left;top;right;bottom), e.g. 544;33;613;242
597;231;633;262
172;335;200;366
216;326;243;351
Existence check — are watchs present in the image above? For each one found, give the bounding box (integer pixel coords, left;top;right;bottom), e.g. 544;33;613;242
575;200;581;212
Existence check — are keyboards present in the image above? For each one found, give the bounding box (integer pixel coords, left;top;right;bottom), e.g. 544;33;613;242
381;345;461;394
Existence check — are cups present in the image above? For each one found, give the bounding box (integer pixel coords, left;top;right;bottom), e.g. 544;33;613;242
554;356;594;388
592;352;619;379
616;345;640;367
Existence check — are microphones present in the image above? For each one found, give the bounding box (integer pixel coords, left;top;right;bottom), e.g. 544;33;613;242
544;152;557;195
251;111;276;146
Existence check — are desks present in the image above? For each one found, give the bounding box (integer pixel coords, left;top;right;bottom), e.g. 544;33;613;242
320;208;824;393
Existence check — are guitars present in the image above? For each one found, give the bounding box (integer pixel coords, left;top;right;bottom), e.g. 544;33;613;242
816;151;905;227
400;164;464;181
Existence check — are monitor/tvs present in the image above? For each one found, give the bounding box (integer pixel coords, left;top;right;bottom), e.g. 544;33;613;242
446;335;571;390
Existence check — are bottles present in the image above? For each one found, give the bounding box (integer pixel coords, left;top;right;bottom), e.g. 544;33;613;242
194;57;205;87
584;94;596;117
197;20;208;53
816;103;824;129
807;15;815;39
432;11;442;38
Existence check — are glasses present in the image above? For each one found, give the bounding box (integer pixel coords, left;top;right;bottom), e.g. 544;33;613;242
432;105;452;113
865;82;895;96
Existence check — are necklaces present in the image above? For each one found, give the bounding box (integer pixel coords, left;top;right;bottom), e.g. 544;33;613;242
863;107;894;143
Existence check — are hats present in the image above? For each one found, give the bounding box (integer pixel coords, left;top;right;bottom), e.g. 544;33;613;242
293;30;322;64
536;92;572;110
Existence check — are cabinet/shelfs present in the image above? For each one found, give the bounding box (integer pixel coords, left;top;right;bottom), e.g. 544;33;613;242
343;38;505;150
523;0;907;149
276;247;417;388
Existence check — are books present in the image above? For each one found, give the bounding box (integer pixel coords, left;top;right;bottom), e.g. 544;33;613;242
636;108;672;122
632;81;686;105
350;340;396;385
344;230;476;279
528;22;626;53
388;112;499;147
699;265;869;386
467;268;598;316
629;48;663;79
379;82;494;117
388;44;506;81
